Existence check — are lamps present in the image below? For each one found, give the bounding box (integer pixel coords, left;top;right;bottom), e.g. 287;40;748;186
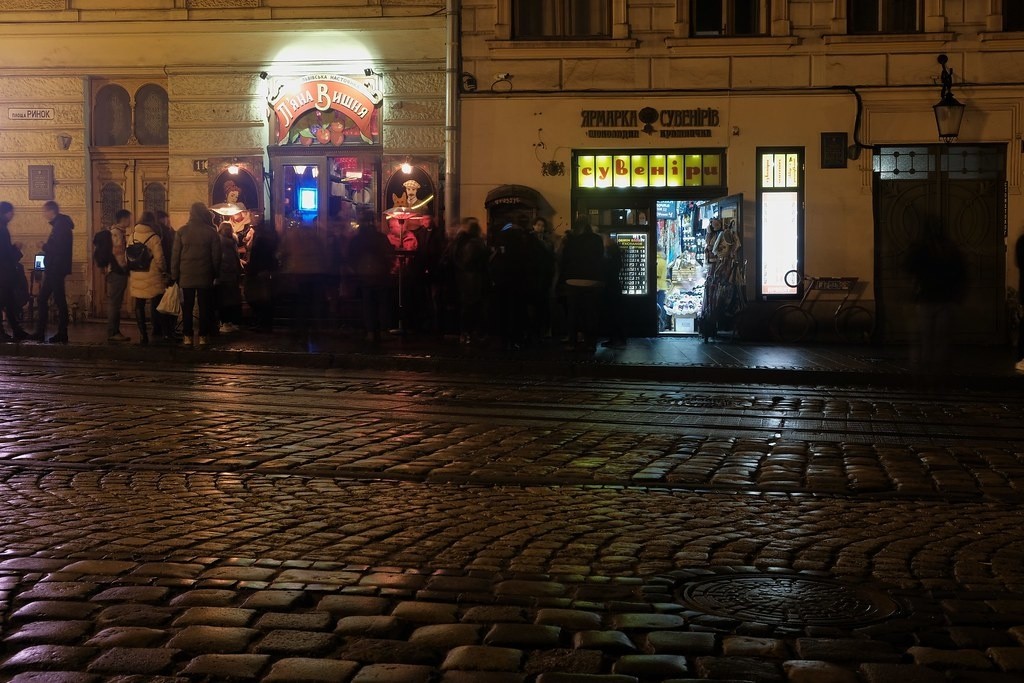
364;68;383;79
932;53;965;145
260;72;270;80
227;157;240;174
399;156;414;174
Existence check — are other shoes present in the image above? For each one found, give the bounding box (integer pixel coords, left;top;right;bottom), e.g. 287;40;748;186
48;334;68;344
140;337;149;346
0;329;13;343
13;329;33;343
365;331;376;343
218;321;240;332
107;333;131;342
199;336;208;345
32;333;45;342
183;335;194;344
380;330;396;340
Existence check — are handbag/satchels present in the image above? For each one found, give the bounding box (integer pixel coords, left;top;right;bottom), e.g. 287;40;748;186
156;282;184;316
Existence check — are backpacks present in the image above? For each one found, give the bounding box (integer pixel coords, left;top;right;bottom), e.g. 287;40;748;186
93;230;112;268
126;232;158;272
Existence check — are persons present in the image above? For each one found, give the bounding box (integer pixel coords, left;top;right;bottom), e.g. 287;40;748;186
902;217;968;359
0;203;630;354
1015;234;1024;369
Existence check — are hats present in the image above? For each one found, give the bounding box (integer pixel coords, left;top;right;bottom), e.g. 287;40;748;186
0;202;12;214
